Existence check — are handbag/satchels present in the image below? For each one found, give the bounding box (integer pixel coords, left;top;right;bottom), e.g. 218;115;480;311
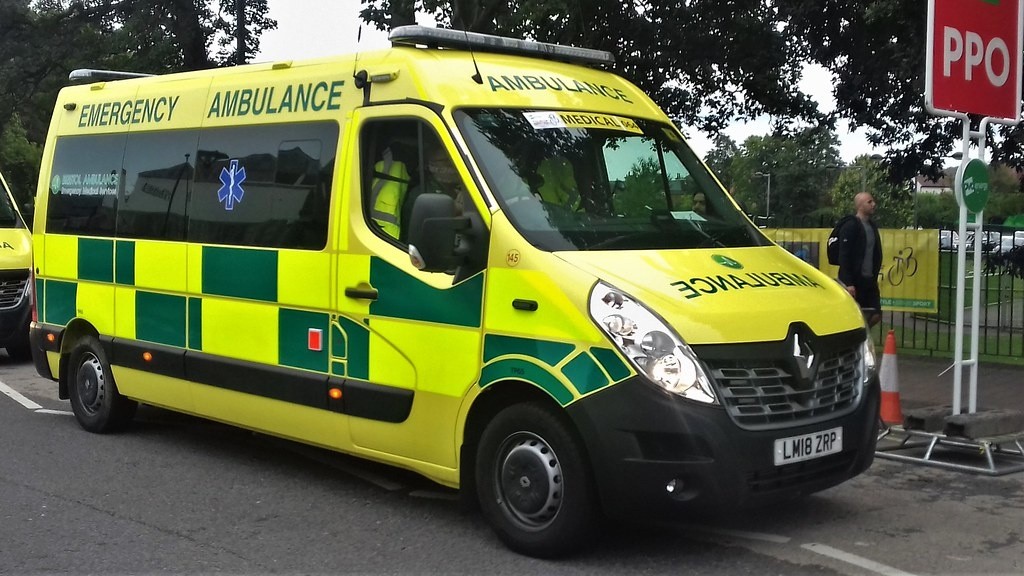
827;215;860;265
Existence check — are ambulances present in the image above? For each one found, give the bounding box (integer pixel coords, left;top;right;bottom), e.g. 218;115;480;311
29;25;881;560
0;174;29;365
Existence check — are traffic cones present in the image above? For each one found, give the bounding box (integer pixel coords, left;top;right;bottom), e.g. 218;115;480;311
877;326;904;427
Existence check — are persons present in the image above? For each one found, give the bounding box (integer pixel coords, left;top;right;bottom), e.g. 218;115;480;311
692;190;706;215
398;144;466;245
838;191;883;329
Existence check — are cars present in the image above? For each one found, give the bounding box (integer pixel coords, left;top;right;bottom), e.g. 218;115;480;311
901;226;1024;252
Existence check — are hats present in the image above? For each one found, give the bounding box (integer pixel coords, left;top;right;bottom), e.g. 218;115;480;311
423;141;448;161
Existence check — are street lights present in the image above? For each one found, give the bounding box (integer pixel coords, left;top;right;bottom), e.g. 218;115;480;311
756;172;771;217
68;69;158;82
